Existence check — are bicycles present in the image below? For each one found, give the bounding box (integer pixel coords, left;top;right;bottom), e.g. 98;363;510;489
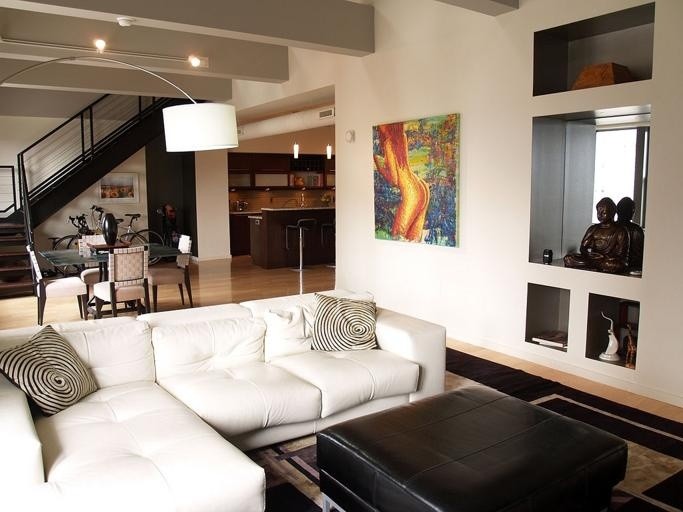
51;205;166;277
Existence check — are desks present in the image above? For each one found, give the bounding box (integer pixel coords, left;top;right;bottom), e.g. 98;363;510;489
38;243;182;314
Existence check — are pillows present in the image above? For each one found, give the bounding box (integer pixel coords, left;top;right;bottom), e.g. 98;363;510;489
309;292;380;351
149;318;266;378
0;324;97;419
57;320;156;388
265;303;314;358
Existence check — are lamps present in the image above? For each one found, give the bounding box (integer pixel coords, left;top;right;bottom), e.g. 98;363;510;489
0;55;238;153
293;132;299;159
95;39;200;68
326;126;332;159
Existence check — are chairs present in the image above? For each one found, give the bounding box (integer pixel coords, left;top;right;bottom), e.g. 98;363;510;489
147;234;193;312
93;245;150;319
27;245;88;326
78;235;109;303
321;219;335;269
286;218;316;271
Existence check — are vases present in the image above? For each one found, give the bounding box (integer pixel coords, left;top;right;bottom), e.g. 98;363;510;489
101;213;118;246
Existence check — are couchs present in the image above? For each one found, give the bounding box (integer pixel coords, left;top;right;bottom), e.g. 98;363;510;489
0;287;445;511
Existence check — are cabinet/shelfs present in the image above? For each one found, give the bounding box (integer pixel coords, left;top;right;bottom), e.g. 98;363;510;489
230;214;258;256
228;152;336;191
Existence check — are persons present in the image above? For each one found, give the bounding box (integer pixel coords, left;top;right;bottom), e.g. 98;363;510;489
563;198;631;273
373;122;430;242
615;198;644;270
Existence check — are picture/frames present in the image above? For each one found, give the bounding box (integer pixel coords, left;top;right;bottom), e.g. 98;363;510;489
96;172;140;204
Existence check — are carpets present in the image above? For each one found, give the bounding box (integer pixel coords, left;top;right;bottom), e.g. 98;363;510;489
245;347;682;511
